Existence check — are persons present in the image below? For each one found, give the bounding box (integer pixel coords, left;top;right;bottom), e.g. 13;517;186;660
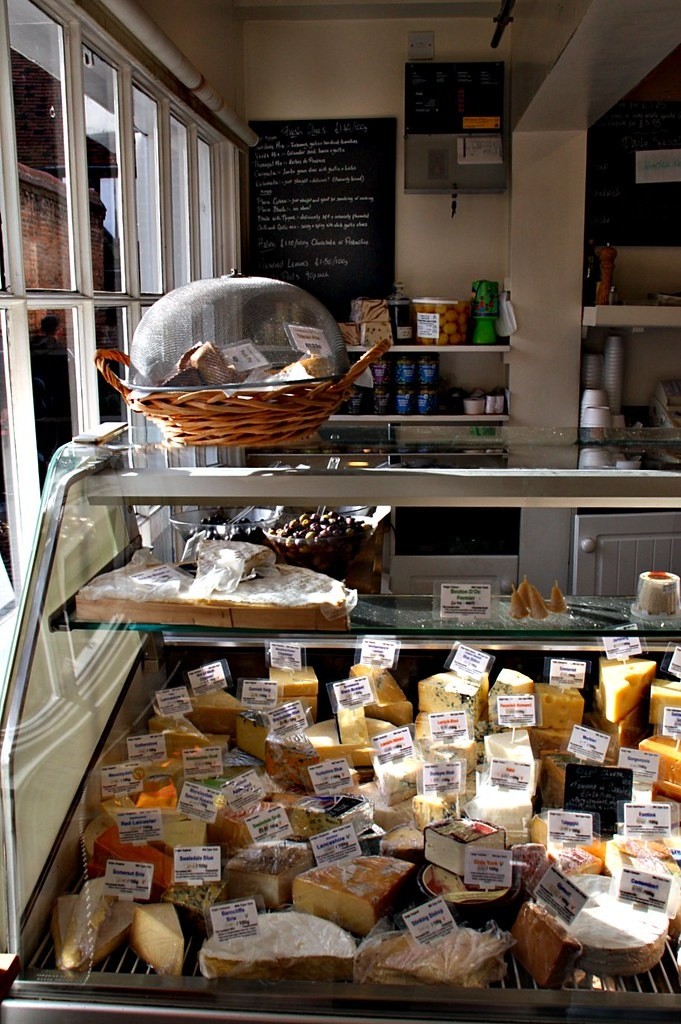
31;316;58;350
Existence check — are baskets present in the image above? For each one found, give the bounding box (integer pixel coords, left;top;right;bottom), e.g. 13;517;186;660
94;338;392;446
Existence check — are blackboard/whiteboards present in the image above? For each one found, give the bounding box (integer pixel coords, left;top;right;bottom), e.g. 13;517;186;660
243;115;399;347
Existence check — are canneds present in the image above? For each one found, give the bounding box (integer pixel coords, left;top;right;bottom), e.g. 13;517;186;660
341;352;440;416
388;300;413;345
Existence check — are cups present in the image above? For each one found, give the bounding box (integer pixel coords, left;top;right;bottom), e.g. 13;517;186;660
577;335;641;470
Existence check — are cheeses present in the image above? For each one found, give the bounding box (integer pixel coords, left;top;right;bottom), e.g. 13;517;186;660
49;655;681;993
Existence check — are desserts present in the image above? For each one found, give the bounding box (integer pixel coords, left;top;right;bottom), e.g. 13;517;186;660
509;573;567;620
636;571;680;616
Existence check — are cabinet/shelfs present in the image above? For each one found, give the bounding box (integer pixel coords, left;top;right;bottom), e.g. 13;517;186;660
567;306;681;599
231;344;524;596
0;422;681;1024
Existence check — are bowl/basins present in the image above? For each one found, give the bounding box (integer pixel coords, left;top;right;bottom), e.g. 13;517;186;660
262;515;378;579
169;508;282;547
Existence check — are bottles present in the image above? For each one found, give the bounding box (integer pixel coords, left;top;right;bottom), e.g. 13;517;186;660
388;282;413;346
584;239;620;306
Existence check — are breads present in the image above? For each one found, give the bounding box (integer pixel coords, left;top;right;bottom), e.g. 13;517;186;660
338;299;394;346
156;340;335;390
74;541;351;629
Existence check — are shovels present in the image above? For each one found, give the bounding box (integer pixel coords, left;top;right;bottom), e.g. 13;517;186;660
227;461;283;524
